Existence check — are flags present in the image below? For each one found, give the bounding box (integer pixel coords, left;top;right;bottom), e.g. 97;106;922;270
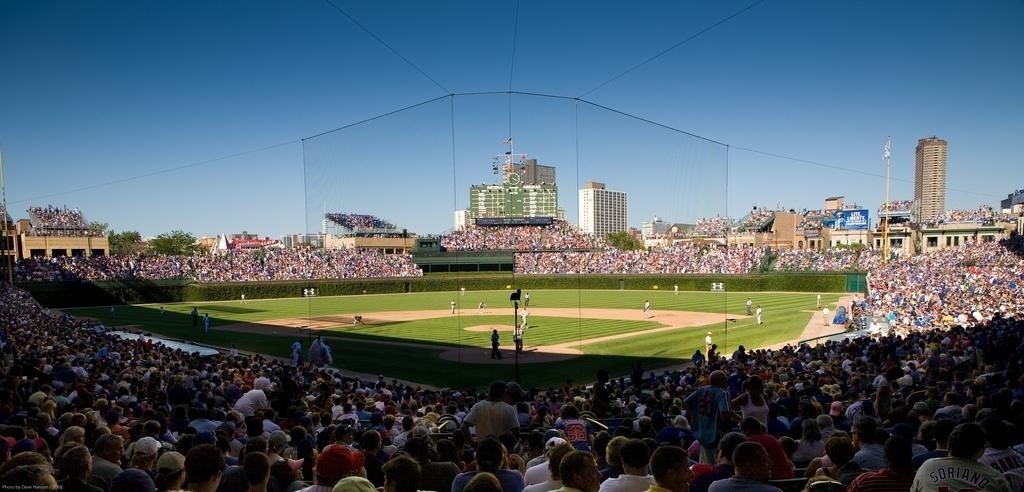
502;136;512;144
882;139;890;160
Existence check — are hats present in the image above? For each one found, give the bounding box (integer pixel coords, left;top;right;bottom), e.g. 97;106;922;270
134;436;186;472
544;429;566;450
254;376;270;389
829;401;843;416
270;425;379;492
412;423;429;438
225;409;245;425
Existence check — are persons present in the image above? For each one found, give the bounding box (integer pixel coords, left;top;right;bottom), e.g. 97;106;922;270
755;305;763;324
0;197;1024;287
520;308;528;328
354;315;363;327
203;312;209;332
524;292;529;306
477;301;486;311
705;332;712;355
450;298;455;314
823;305;830;326
102;335;1024;492
817;293;821;308
0;287;103;491
490;330;502;360
745;297;752;314
512;324;524;352
644;300;650;317
191;306;198;325
240;292;246;304
834;288;1023;335
159;306;165;318
